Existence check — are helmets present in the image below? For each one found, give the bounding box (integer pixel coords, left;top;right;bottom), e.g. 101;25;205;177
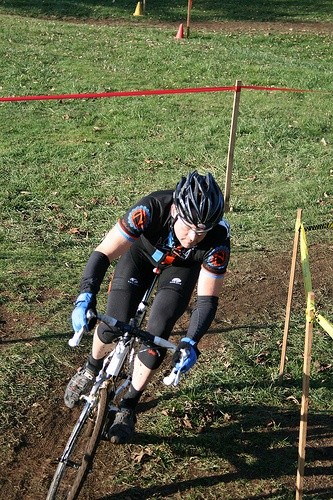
173;169;224;230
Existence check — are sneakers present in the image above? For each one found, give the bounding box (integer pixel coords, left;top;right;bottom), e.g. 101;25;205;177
64;359;99;409
107;398;136;444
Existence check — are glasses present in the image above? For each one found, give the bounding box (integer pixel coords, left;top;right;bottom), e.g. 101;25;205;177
176;215;213;235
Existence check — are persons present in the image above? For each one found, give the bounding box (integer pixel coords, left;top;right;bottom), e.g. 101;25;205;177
65;170;231;446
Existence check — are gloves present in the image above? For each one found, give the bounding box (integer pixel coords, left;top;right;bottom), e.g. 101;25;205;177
71;293;97;333
163;337;197;386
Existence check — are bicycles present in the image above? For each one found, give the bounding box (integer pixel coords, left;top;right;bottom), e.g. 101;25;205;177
46;268;191;500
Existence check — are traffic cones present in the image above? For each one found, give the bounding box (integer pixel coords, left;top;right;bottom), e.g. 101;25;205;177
133;1;144;16
175;23;183;38
